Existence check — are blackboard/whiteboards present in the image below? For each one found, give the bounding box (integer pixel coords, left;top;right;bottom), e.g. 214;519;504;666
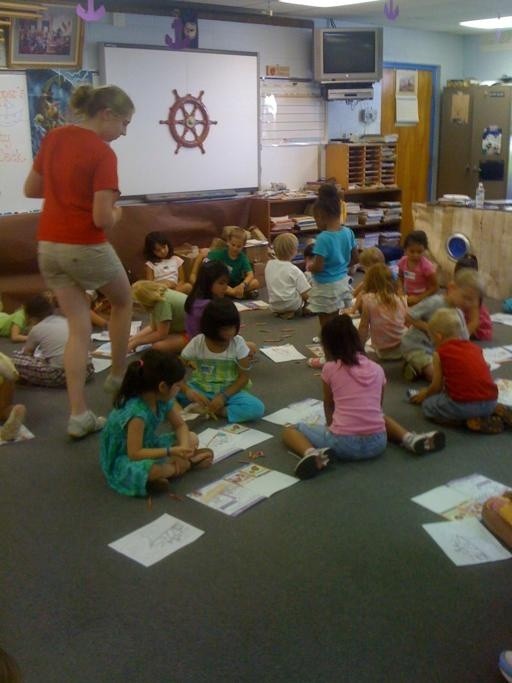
96;40;262;203
0;68;47;216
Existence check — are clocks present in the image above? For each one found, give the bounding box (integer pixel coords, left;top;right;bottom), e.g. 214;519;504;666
165;7;199;50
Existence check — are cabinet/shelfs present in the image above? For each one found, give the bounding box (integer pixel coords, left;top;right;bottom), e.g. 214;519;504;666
252;192;323;268
317;188;404;249
435;80;512;197
327;140;400;187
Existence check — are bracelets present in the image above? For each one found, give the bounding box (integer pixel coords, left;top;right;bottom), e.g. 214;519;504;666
192;271;198;275
240;282;248;288
167;448;171;457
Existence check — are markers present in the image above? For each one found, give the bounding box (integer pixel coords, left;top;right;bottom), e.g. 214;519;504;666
0;208;41;215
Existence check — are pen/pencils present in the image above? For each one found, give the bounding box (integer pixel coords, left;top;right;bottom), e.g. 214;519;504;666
259;329;272;332
280;334;291;337
234;459;250;463
205;407;218;420
280;328;295;331
263;339;280;342
256;322;267;325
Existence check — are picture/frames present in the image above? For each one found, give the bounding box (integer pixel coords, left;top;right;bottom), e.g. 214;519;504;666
6;1;89;71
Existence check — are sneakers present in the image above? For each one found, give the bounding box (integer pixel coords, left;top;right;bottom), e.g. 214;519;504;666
307;356;326;369
246;289;259;299
493;404;512;429
293;446;337;482
0;403;27;442
67;409;107;439
463;414;505;435
402;360;424;383
149;477;169;494
407;431;446;457
102;373;124;394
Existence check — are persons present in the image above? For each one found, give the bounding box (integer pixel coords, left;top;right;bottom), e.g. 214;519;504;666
22;83;133;440
1;259;266;498
264;186;512;480
143;231;192;294
189;227;259;299
499;650;512;683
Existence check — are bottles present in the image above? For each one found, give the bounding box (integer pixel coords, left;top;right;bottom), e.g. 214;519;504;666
475;182;486;208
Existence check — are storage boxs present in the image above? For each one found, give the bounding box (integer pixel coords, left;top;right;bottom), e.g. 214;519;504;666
173;240;268;289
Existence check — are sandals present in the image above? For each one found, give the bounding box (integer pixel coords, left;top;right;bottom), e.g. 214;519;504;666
185;447;214;473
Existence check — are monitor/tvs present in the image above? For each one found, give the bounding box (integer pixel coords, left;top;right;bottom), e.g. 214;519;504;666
313;25;384;83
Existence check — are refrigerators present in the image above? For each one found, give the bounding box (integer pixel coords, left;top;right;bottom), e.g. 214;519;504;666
437;84;511;200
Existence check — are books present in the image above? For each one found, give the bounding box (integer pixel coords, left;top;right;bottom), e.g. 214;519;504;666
234;302;250;312
494;378;511;405
305;343;326;357
91;356;112;374
90;341;152;358
186;462;301;517
261;343;305;362
488;312;511;326
307;357;327;367
255;190;401;261
242;299;270;309
90;332;137;341
263;398;327;427
195;423;274;465
485;347;510;364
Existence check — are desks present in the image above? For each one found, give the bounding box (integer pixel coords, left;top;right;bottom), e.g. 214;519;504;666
404;194;512;309
